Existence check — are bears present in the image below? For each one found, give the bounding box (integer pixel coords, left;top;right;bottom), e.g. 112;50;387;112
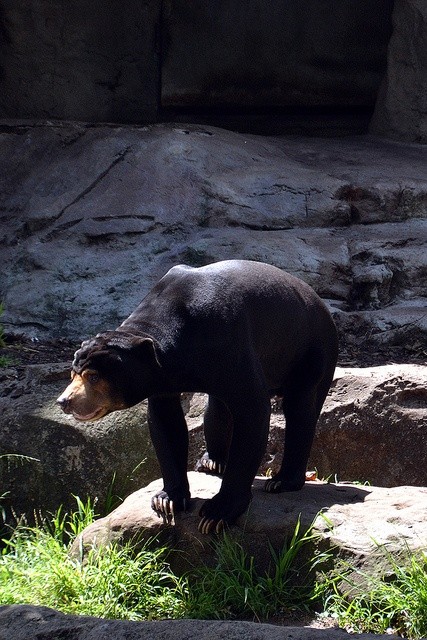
58;257;340;538
